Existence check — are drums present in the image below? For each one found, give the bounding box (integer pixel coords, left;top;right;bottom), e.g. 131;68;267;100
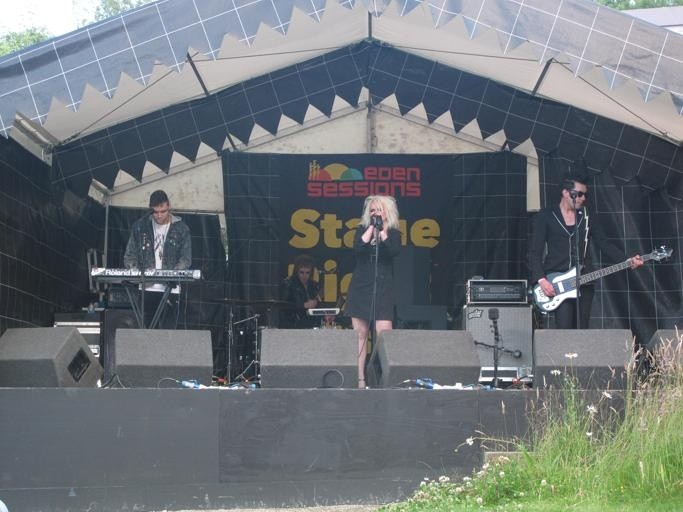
223;305;251;329
254;311;268;330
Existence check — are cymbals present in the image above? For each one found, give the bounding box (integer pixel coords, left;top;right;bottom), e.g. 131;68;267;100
211;298;251;303
252;299;289;304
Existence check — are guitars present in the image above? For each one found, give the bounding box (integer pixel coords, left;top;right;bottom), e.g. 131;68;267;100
533;245;674;312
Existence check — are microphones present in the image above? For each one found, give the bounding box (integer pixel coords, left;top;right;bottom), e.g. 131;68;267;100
571;191;578;200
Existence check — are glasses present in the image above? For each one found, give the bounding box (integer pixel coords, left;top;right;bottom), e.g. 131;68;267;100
566;189;588;198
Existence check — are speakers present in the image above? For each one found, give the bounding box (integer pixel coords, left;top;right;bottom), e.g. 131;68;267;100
636;328;683;391
460;301;533;387
261;329;359;388
365;329;480;388
533;329;636;390
110;328;213;389
0;327;103;387
104;309;150;385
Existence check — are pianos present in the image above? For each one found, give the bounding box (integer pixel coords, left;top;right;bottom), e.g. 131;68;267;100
91;267;207;287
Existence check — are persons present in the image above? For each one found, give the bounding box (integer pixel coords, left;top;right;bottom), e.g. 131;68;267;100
123;190;193;329
323;291;354;329
270;254;321;329
342;194;401;390
527;175;644;329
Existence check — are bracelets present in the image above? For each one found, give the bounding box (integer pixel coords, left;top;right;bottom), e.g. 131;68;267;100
536;277;545;285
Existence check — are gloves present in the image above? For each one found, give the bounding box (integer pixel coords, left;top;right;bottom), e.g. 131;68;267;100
369;212;384;231
